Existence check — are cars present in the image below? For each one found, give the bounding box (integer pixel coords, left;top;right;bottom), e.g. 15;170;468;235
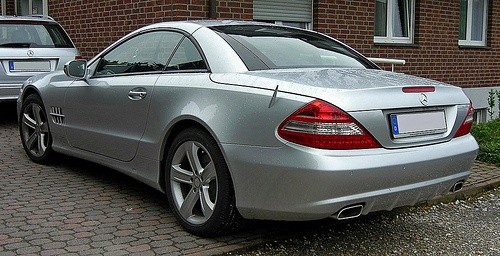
0;13;83;101
15;19;481;236
